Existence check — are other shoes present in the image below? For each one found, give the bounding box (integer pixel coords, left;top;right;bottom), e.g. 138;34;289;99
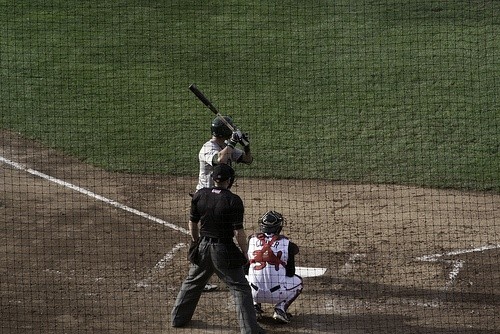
203;284;217;292
272;308;289;323
254;304;262;313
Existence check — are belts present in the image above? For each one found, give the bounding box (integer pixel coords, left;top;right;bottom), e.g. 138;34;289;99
202;236;218;244
251;283;281;293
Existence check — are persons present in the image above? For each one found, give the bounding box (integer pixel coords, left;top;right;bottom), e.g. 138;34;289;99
244;210;304;322
170;164;266;334
196;117;254;292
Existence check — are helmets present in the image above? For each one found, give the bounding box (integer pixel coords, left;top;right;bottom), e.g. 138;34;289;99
258;210;284;233
211;116;235;139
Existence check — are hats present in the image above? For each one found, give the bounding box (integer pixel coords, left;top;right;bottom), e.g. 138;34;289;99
213;164;232;183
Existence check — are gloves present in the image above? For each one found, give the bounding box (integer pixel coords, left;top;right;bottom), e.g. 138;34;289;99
226;130;250;152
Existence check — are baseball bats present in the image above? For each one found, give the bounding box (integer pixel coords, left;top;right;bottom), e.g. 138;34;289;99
189;84;251;148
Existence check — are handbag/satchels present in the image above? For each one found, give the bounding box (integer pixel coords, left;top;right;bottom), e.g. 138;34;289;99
187;237;202;264
225;241;250;278
